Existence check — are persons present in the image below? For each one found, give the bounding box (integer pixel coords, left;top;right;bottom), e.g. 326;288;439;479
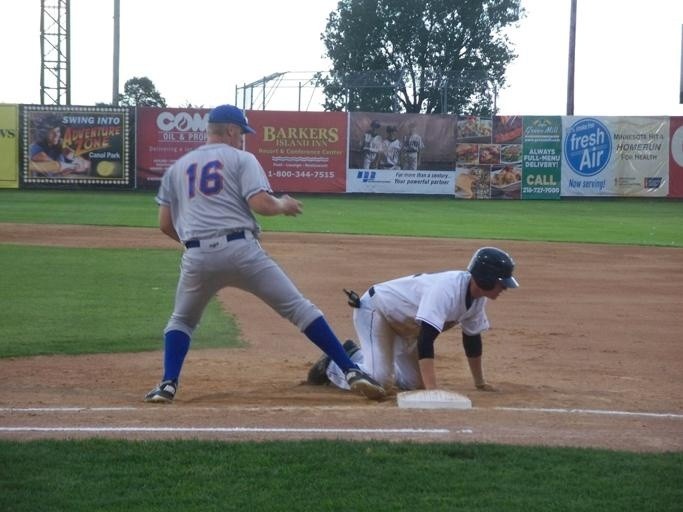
29;112;91;177
357;120;384;170
377;122;402;171
401;122;425;171
139;104;390;404
307;247;520;392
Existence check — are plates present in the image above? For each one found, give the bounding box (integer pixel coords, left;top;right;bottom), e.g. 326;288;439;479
457;116;521;199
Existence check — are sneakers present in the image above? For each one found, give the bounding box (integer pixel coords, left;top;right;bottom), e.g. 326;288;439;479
344;369;386;399
143;378;178;403
306;337;361;384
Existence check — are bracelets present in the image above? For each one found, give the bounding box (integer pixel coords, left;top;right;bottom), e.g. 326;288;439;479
474;382;487;389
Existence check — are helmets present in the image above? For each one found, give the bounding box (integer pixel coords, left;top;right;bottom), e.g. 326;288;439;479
469;246;522;290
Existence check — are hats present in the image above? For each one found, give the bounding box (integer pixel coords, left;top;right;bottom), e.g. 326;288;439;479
207;104;257;134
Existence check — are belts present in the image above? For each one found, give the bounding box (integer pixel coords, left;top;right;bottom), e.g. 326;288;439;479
367;283;378;301
184;229;261;249
